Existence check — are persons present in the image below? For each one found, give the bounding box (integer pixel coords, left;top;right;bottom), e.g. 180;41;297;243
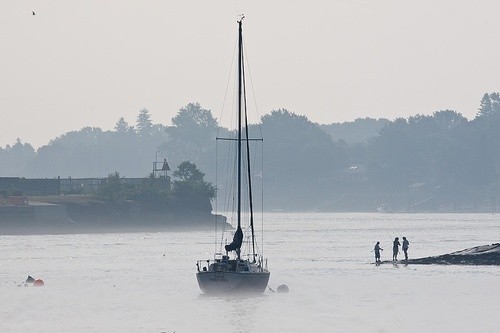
371;241;385;262
392;237;401;261
402;237;410;259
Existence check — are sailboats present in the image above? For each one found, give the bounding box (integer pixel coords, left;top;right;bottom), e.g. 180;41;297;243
192;14;272;297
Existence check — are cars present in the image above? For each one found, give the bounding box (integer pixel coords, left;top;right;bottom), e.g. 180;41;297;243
1;188;29;206
